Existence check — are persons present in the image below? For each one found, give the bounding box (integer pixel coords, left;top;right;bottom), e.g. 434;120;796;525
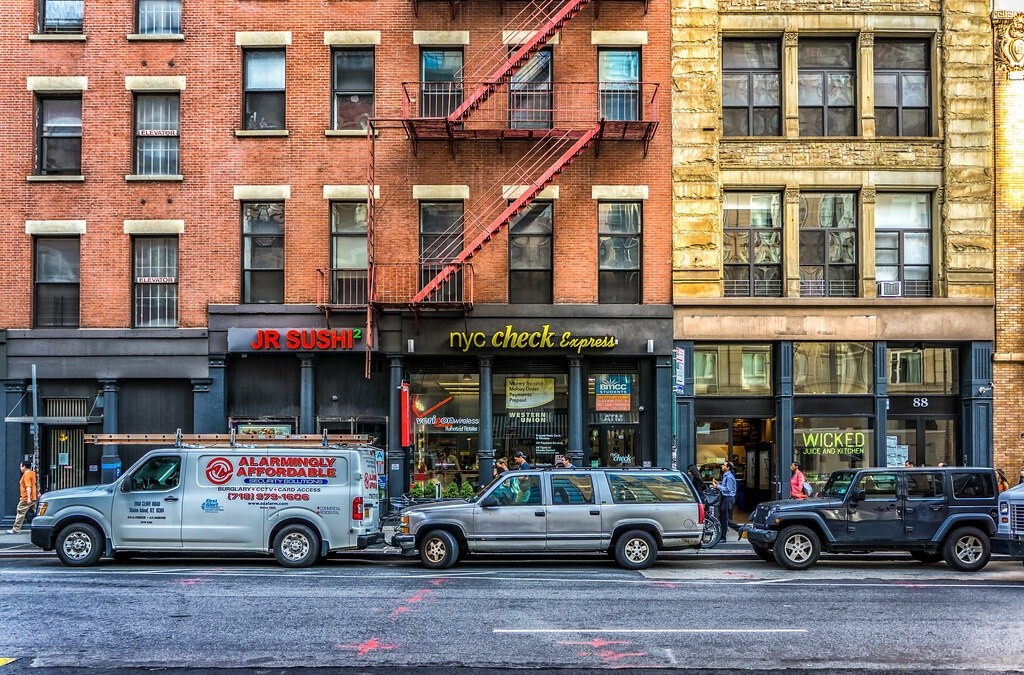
995;461;1024;494
440;447;461;471
686;465;710;511
7;461;37;534
713;462;746;542
492;450;576;503
905;459;948;467
788;462;805;499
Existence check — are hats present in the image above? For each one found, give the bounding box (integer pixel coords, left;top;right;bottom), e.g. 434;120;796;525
514;451;526;458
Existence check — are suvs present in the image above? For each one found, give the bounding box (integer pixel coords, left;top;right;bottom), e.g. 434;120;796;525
997;479;1024;564
392;465;707;569
737;466;1001;573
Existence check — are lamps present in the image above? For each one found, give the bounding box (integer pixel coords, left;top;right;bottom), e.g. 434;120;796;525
647;339;654;352
408;339;415;352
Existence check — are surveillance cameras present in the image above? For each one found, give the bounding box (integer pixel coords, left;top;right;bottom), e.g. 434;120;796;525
989;381;994;389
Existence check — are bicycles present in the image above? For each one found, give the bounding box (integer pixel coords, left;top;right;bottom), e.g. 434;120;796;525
381;494;417;547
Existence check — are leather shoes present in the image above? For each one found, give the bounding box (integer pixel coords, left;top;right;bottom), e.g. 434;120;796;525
738;527;744;541
719;540;726;543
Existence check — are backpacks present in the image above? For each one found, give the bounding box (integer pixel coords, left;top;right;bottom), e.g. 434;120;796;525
511;477;521;494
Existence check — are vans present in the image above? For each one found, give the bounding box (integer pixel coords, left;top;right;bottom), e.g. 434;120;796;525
29;427;389;569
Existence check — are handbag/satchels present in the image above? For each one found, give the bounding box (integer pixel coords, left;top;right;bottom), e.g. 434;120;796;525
702;487;720;506
802;481;813;497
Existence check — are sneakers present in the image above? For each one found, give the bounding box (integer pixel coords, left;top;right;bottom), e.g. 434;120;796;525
6;529;19;533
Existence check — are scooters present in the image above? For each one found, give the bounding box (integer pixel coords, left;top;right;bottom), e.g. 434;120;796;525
701;487;725;549
